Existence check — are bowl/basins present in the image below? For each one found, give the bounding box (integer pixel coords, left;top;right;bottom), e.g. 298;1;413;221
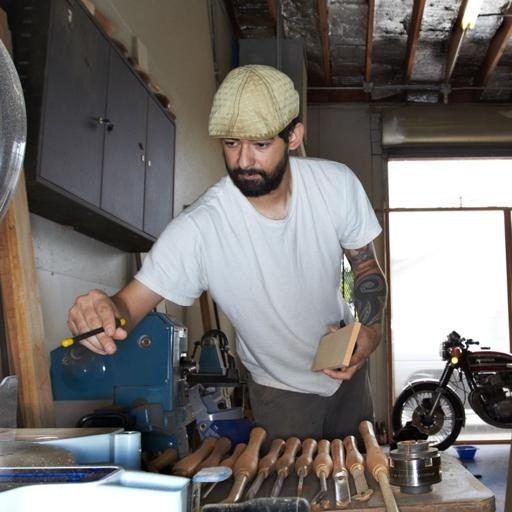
454;446;478;459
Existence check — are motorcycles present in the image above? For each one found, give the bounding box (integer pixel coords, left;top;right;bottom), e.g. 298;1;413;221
392;331;512;451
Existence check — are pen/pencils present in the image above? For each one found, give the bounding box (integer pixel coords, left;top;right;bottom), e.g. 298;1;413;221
340;320;355;356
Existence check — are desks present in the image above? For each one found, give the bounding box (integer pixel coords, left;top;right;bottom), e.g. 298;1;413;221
186;442;498;511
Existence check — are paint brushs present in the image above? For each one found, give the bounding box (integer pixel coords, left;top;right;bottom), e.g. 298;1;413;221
62;318;126;348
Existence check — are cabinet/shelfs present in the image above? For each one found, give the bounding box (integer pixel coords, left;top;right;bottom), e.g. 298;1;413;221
143;90;176;252
8;0;147;253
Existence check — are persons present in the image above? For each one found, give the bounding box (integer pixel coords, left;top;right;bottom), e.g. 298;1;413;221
67;63;390;452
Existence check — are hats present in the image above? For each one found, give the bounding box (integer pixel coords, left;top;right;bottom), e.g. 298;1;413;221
208;63;300;141
